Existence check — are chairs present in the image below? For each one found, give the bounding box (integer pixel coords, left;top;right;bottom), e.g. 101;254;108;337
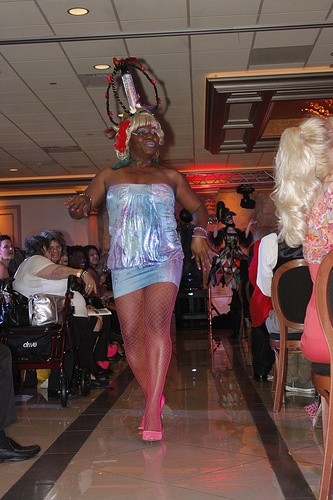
0;239;333;500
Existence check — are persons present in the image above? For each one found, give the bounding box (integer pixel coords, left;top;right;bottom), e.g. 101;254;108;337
206;201;257;334
0;229;123;464
64;111;210;443
246;115;333;430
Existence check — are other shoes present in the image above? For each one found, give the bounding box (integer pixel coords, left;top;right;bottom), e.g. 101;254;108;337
254;373;270;382
90;372;109;388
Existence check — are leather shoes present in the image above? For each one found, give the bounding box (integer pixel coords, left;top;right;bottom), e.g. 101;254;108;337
0;437;41;462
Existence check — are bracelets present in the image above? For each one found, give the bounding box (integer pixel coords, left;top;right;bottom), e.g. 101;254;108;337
80;270;88;278
79;193;92;205
193;227;208;236
192;234;207;239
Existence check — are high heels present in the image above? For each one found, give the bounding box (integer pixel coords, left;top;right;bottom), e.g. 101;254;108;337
137;394;165;442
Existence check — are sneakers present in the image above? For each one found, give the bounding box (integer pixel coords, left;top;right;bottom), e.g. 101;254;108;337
285;377;315;394
286;392;316;407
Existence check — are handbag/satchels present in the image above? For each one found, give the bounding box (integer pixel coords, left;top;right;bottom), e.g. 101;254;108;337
29;293;65;327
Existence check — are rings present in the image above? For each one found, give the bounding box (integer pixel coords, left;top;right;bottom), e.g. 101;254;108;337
87;285;91;288
